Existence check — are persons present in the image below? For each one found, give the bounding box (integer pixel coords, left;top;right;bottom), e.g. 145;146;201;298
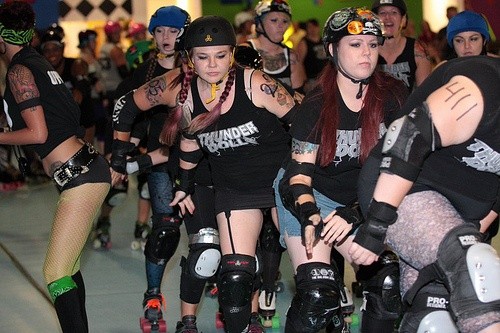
0;0;500;333
109;19;302;333
0;0;117;332
356;33;500;332
273;9;408;333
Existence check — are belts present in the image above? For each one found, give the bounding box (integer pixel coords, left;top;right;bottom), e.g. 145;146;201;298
54;141;97;190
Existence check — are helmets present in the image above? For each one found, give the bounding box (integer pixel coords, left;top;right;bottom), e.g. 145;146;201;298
148;6;191;35
45;23;64;35
369;0;408;29
447;11;489;48
79;30;97;43
39;32;63;50
322;7;384;56
255;0;292;25
129;23;145;35
184;15;236;48
104;22;120;33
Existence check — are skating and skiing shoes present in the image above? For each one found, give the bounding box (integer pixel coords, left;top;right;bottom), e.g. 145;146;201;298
215;312;223;327
92;220;112;249
258;308;279;329
341;285;359;327
176;315;199;333
131;223;151;252
248;325;264;333
139;288;167;333
